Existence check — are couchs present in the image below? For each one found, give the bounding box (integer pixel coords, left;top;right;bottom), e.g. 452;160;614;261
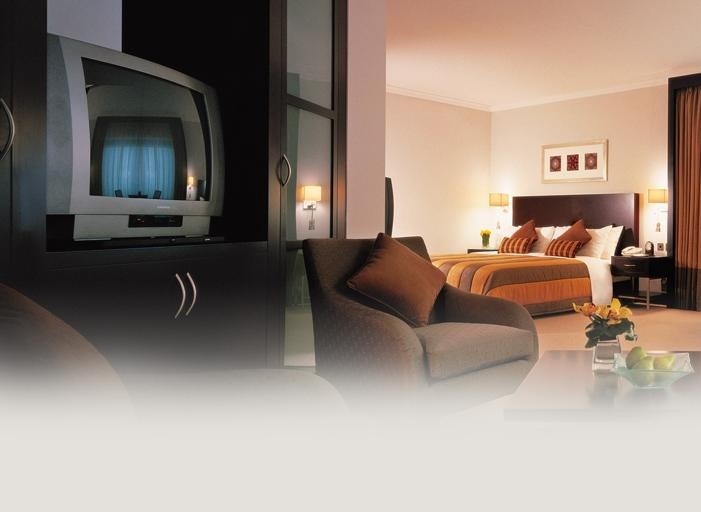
303;235;540;413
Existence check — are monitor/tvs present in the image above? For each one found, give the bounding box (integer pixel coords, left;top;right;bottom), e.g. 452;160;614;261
45;30;227;241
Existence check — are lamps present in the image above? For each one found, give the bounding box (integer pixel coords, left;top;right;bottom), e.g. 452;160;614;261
489;192;511;215
300;185;325;231
649;185;669;213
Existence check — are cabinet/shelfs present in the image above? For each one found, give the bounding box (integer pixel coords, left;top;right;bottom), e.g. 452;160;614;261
277;2;347;372
41;243;268;420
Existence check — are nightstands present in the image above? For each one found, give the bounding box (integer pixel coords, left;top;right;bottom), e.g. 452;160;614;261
467;247;497;254
612;256;669;311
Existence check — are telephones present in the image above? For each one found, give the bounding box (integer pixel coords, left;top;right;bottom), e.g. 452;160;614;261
621;245;643;256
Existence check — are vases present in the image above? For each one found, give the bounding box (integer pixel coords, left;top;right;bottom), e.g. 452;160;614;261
482;239;489;248
591;342;620;386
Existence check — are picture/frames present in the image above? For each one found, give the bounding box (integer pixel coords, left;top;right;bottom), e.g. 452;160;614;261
540;138;609;187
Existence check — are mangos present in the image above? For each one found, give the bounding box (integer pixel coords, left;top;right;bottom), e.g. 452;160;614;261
652;354;675;371
631;356;655;383
626;346;647;369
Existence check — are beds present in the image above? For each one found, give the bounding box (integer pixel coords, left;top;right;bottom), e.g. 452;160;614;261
430;192;640;319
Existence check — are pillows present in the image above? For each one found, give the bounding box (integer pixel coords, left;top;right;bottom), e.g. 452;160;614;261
497;236;534;254
554;226;610;258
502;227;554;253
602;224;626;259
545;239;580;258
554;220;591;250
346;232;448;328
510;219;538;245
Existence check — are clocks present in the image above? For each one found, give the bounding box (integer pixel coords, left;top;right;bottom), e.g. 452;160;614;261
644;241;654;254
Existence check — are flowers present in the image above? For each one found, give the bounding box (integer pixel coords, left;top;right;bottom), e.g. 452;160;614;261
481;230;491;245
571;298;638;349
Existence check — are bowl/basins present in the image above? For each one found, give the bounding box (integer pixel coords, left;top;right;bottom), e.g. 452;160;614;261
610;352;695;389
